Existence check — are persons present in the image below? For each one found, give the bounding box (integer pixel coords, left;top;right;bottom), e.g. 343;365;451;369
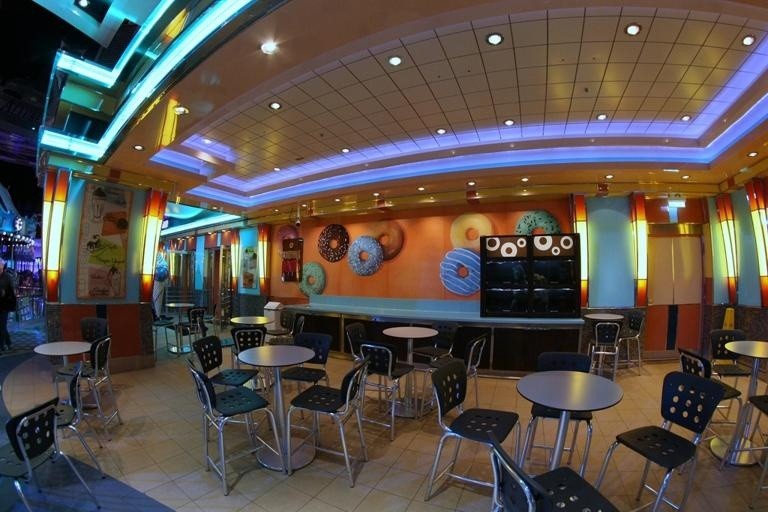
0;258;14;353
4;263;44;298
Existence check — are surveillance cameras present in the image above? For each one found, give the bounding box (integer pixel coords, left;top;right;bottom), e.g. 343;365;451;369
295;220;301;226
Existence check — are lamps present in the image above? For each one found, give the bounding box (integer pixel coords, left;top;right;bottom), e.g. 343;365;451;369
153;91;180;155
666;195;691;212
137;188;168;304
627;193;650;308
744;173;768;309
714;192;740;303
569;193;590;309
229;228;240;290
257;223;271;297
42;169;69;305
